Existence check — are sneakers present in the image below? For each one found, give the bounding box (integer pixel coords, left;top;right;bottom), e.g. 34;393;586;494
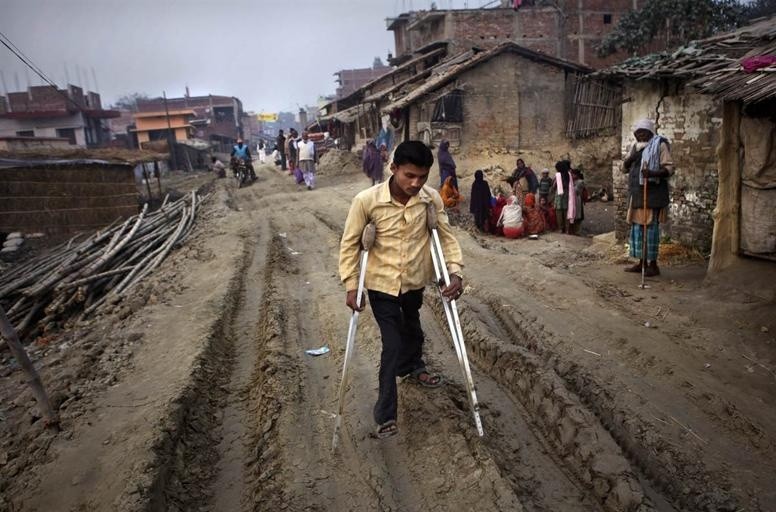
644;266;659;276
624;265;647;272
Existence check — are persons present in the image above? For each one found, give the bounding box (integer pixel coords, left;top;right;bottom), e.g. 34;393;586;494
336;139;464;439
439;175;464;214
469;158;588;240
256;139;266;164
619;119;674;277
362;137;383;185
379;142;388;162
438;139;458;190
210;157;226;178
271;127;320;190
230;139;259;181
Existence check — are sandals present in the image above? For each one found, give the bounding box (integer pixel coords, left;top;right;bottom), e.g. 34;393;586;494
376;420;399;437
403;367;444;388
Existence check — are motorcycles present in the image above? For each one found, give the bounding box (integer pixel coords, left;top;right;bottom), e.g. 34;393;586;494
229;158;254;187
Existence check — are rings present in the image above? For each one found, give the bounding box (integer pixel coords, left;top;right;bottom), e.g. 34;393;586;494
457;291;461;296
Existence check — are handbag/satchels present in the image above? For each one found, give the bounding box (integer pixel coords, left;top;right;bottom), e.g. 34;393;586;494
295;168;304;184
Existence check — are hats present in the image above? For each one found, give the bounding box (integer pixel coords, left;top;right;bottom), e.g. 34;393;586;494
633;119;655;135
